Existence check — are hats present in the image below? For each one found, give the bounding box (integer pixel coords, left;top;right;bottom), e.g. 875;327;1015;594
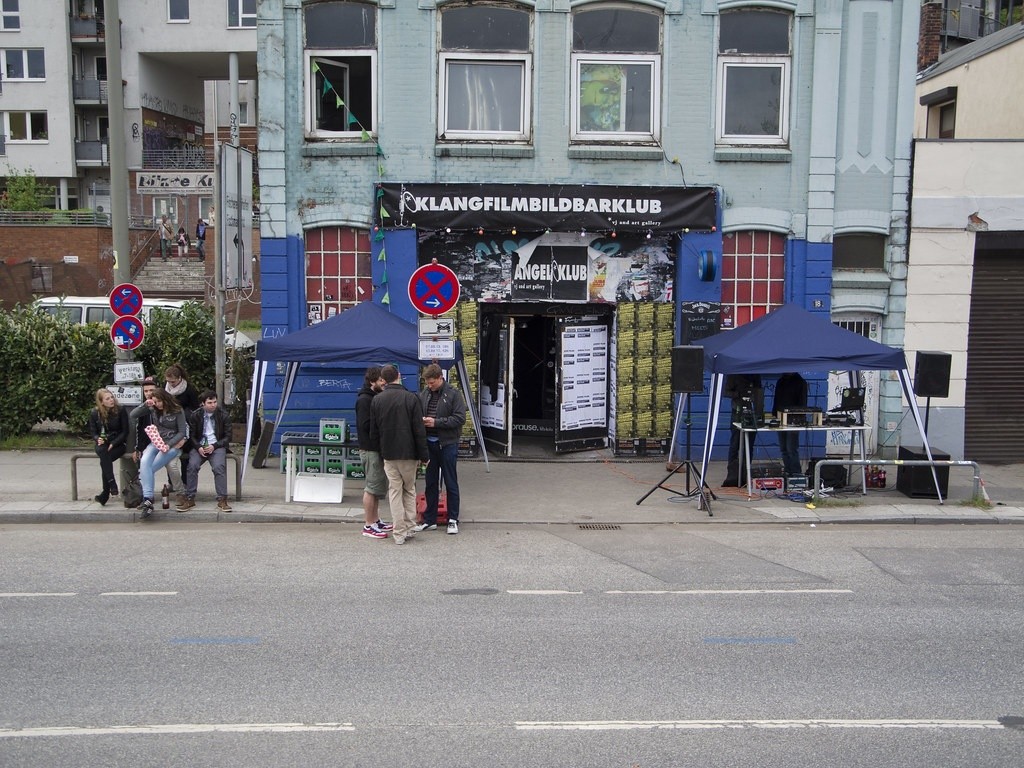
138;376;160;387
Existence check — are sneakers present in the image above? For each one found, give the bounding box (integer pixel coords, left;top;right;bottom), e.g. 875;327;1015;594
363;522;388;538
414;522;438;531
447;519;458;534
177;495;196;511
217;497;232;511
373;518;393;531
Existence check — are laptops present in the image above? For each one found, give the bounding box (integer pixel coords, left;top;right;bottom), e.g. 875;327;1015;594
832;387;866;411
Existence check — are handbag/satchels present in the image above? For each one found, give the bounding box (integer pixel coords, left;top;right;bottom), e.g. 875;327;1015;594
121;470;143;508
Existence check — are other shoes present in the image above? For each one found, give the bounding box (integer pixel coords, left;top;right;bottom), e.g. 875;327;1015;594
161;485;175;493
95;492;110;505
110;479;118;495
137;498;154;520
407;531;416;537
183;484;188;491
175;494;187;506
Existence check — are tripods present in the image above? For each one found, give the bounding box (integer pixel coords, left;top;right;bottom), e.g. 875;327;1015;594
636;393;717;516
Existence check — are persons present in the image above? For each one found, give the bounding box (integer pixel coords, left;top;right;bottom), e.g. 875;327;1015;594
129;358;200;519
195;218;209;261
415;365;466;534
207;206;216;225
354;367;392;537
87;387;129;505
371;363;429;545
176;389;233;512
158;214;174;262
770;371;808;475
176;227;191;266
721;373;765;486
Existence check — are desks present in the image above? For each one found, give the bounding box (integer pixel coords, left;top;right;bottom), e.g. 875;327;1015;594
281;438;360;502
733;422;866;496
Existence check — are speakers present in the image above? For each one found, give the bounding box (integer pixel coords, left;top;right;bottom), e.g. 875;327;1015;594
672;345;704;393
898;445;950;499
913;350;952;398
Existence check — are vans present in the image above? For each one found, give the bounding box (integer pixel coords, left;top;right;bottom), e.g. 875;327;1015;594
32;296;256;358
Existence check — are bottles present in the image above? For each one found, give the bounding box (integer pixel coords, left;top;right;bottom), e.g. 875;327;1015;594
346;423;350;441
100;425;106;445
203;436;210;455
865;465;886;488
162;484;170;509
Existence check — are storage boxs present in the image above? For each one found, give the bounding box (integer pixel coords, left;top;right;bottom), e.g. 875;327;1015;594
294;472;344;503
280;418;365;479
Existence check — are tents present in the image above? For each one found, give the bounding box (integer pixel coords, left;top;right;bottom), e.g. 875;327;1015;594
668;301;945;509
241;299;490;492
